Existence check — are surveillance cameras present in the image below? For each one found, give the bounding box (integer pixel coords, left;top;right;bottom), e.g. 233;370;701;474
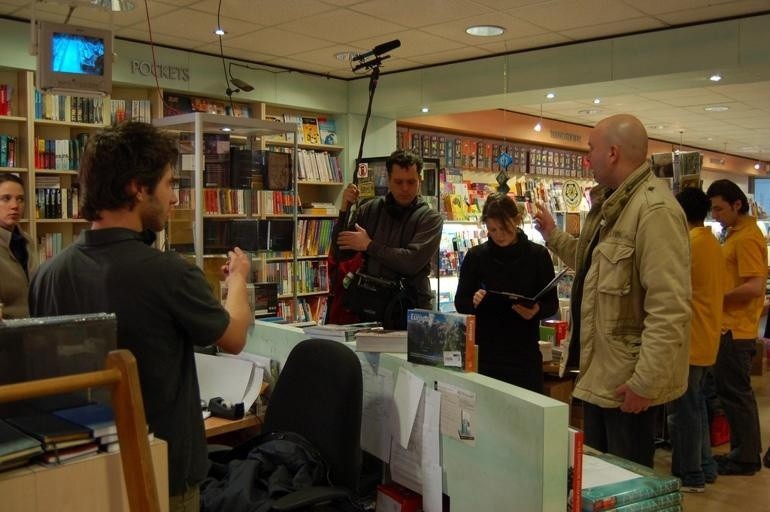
231;79;254;92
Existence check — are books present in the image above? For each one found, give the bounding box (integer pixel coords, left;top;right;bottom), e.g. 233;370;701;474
565;423;683;512
649;149;705;197
485;264;570;309
303;308;479;372
0;82;343;327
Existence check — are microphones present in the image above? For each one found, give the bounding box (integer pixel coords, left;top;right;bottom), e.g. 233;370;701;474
353;39;401;61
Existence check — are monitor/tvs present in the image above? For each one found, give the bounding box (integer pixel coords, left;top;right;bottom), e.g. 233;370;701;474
36;20;113;97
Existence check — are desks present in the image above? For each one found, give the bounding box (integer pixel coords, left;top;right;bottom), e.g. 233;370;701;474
205;397;268;445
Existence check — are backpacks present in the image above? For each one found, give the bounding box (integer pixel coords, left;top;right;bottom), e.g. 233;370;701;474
263;335;369;485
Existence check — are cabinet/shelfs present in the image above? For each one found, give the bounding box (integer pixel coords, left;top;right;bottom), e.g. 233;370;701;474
396;121;599;313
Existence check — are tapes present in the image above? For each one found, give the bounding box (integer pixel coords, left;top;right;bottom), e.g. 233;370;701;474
221;400;231;408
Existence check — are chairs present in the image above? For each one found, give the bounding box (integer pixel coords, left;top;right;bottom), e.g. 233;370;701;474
201;338;379;512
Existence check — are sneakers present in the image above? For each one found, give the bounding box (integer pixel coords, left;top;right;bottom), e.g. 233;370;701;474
715;450;762;475
673;468;707;494
700;458;720;483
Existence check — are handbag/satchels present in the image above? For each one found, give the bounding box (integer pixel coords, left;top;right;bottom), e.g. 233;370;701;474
342;271;441;328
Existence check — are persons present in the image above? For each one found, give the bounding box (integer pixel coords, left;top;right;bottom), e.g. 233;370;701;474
331;149;441;332
452;193;558;395
25;121;252;511
671;186;727;493
532;113;691;473
707;180;767;476
